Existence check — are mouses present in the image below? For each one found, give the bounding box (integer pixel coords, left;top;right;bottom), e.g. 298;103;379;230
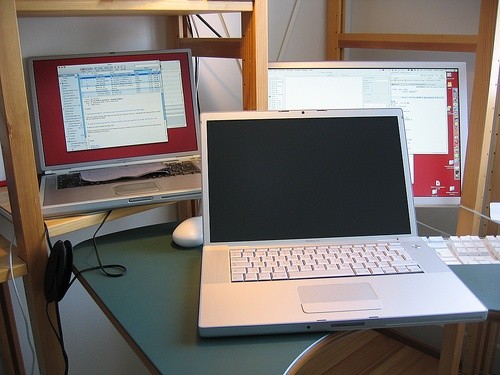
172;216;203;247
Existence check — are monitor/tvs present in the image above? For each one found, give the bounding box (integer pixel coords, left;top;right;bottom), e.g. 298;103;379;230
269;61;469;208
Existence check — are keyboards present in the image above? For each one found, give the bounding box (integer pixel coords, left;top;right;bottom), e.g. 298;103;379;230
420;235;500;266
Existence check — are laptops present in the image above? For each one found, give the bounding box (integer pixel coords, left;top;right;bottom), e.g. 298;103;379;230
28;48;203;219
199;108;488;337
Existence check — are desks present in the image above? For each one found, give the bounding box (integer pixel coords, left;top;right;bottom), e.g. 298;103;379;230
70;204;500;375
